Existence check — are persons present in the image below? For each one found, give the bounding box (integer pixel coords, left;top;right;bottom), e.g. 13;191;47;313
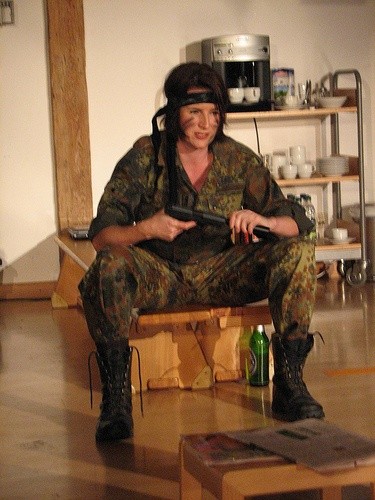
77;61;325;443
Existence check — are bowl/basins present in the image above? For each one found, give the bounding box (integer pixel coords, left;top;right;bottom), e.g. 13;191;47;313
317;96;348;108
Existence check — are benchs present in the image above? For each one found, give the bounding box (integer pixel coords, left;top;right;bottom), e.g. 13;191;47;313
52;233;272;389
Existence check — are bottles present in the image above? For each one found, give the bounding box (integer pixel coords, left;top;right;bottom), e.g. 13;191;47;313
286;193;318;241
247;324;270;386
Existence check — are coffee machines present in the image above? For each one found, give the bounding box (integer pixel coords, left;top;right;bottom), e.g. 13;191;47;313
201;33;271;113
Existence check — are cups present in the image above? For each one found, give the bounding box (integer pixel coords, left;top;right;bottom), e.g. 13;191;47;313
227;88;244;104
244;86;260;104
331;228;349;240
283;95;298;107
265;145;312;180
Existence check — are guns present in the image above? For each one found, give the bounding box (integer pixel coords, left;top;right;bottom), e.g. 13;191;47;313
163;203;271;238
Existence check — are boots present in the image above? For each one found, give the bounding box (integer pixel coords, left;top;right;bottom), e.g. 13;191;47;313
94;339;136;446
270;334;325;420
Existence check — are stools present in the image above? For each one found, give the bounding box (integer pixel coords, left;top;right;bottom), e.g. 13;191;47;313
178;430;375;500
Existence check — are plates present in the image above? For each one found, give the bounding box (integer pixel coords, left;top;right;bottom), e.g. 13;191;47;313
316;157;351;177
325;237;356;245
275;106;305;110
